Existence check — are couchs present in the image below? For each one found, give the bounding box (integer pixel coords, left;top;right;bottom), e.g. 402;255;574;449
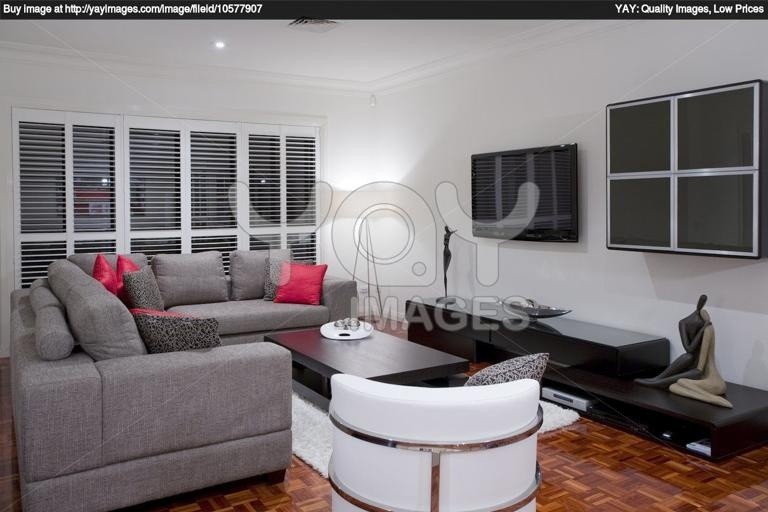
9;274;359;512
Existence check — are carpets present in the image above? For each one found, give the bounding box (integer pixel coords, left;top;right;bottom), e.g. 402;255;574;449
291;373;581;477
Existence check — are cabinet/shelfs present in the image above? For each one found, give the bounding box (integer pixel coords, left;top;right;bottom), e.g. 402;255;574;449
605;79;768;259
404;300;768;464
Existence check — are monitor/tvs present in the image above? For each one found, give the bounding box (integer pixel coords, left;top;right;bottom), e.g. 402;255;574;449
470;142;578;243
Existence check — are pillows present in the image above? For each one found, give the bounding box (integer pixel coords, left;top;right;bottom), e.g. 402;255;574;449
48;250;328;360
466;352;549;384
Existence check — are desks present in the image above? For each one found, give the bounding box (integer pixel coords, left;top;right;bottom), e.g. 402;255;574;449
265;330;470;401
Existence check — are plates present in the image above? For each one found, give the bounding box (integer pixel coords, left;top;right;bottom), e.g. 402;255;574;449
498;301;573;324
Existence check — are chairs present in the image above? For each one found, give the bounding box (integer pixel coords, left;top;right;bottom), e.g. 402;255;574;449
328;374;544;512
